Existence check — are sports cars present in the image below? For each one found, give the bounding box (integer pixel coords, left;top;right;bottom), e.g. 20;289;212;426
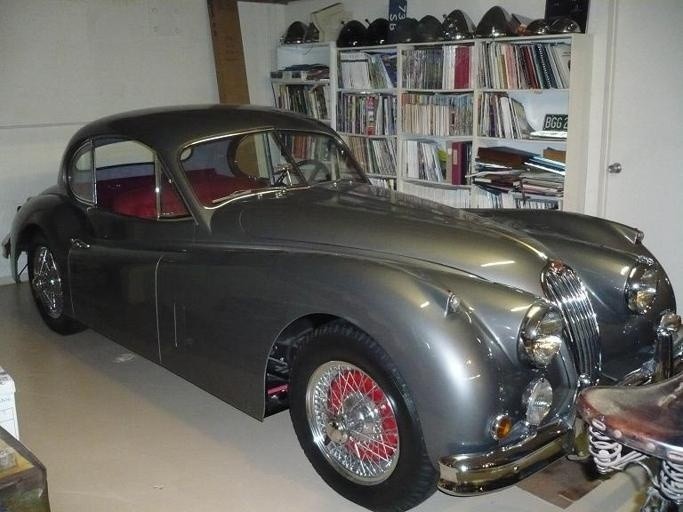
3;103;682;511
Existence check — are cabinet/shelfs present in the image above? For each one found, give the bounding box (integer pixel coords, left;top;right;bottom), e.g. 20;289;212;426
268;32;594;216
